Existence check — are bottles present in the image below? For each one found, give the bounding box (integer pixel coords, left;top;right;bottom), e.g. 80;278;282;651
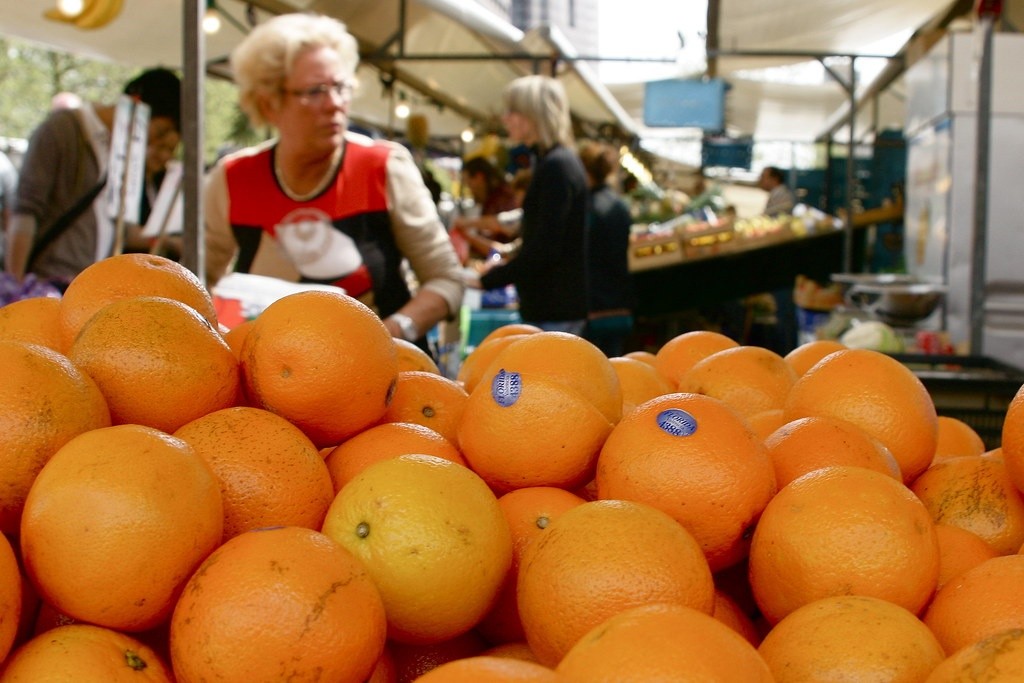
484;248;506;308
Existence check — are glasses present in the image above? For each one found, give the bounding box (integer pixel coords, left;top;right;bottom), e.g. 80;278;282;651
278;77;357;106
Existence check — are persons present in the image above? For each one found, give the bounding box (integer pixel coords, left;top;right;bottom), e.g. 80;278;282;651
0;68;184;298
395;74;796;359
200;12;466;367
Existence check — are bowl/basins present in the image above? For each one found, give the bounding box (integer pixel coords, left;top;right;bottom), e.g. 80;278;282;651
830;272;946;323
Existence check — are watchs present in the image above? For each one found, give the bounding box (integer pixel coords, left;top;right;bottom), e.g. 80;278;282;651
391;312;420;343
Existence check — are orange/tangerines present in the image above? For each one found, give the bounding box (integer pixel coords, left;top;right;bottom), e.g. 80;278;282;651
0;255;1024;683
